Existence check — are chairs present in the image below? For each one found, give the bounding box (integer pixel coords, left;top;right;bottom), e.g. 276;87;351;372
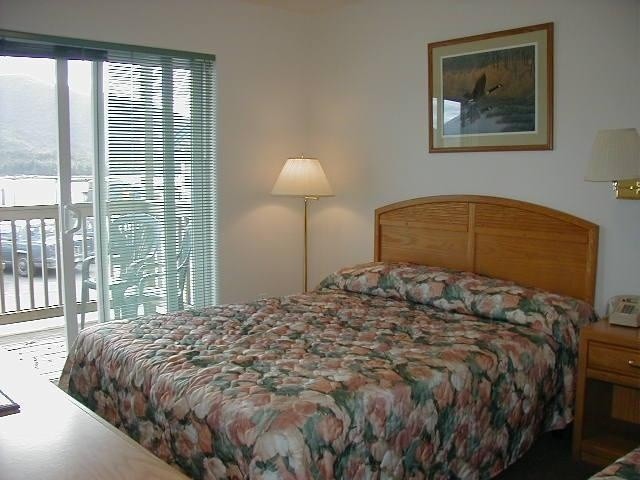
77;210;164;332
120;229;191;322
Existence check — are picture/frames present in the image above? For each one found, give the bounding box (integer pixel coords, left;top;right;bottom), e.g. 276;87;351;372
425;20;556;157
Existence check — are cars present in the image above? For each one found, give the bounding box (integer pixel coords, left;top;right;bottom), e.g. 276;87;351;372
1;173;168;276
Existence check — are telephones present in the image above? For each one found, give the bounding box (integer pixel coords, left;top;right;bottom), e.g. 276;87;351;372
607;294;640;328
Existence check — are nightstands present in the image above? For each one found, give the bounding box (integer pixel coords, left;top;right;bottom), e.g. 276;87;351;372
567;318;639;467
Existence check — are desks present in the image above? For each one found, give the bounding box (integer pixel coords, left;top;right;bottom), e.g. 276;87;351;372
1;351;192;479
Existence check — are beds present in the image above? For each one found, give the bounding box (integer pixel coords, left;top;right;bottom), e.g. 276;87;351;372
57;193;602;478
584;447;640;480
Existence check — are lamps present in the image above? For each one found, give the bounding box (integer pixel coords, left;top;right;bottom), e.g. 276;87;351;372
577;125;640;202
269;150;337;293
